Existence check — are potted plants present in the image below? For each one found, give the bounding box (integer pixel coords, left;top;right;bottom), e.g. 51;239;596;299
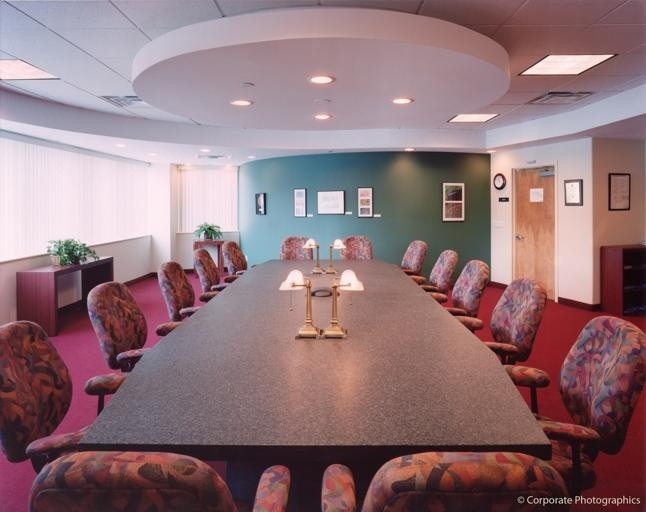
193;222;223;241
47;237;99;266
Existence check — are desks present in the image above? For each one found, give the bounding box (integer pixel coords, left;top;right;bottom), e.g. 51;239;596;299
16;256;114;336
192;239;225;277
77;258;552;512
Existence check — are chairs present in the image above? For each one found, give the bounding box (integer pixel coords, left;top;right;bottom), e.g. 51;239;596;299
158;262;220;321
339;235;373;261
221;241;257;275
503;315;646;500
409;249;459;295
426;259;489;315
281;237;313;259
453;276;547;365
1;320;128;475
321;451;571;512
26;451;291;511
400;240;429;276
86;280;185;373
192;248;238;293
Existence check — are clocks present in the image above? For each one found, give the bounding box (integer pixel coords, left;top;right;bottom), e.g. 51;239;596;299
493;173;506;190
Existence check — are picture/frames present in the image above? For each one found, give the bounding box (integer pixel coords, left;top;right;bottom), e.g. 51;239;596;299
441;181;466;223
609;172;631;210
293;188;306;217
316;190;345;215
564;179;582;206
255;194;266;215
357;186;373;218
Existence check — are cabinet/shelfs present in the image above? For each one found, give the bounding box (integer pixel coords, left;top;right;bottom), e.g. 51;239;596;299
600;244;645;315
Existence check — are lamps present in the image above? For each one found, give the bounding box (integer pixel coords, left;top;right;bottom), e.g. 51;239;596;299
319;239;345;274
279;269;324;340
321;268;366;339
303;238;325;275
